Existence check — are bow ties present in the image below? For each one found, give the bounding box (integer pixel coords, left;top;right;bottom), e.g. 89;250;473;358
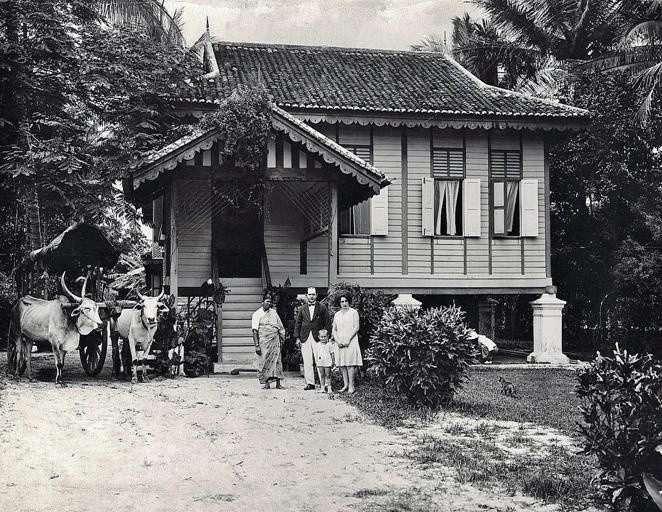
308;304;315;307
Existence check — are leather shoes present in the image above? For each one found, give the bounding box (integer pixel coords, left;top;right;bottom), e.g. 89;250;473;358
304;384;316;391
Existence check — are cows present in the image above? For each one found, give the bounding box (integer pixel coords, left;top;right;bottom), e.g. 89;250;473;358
109;285;167;385
12;269;105;389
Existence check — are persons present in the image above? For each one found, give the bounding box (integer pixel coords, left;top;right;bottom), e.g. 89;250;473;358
70;276;92;302
333;290;363;393
252;294;285;388
294;286;332;390
469;331;499;364
314;330;333;394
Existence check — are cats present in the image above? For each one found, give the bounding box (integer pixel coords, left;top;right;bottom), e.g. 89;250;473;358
497;376;521;400
